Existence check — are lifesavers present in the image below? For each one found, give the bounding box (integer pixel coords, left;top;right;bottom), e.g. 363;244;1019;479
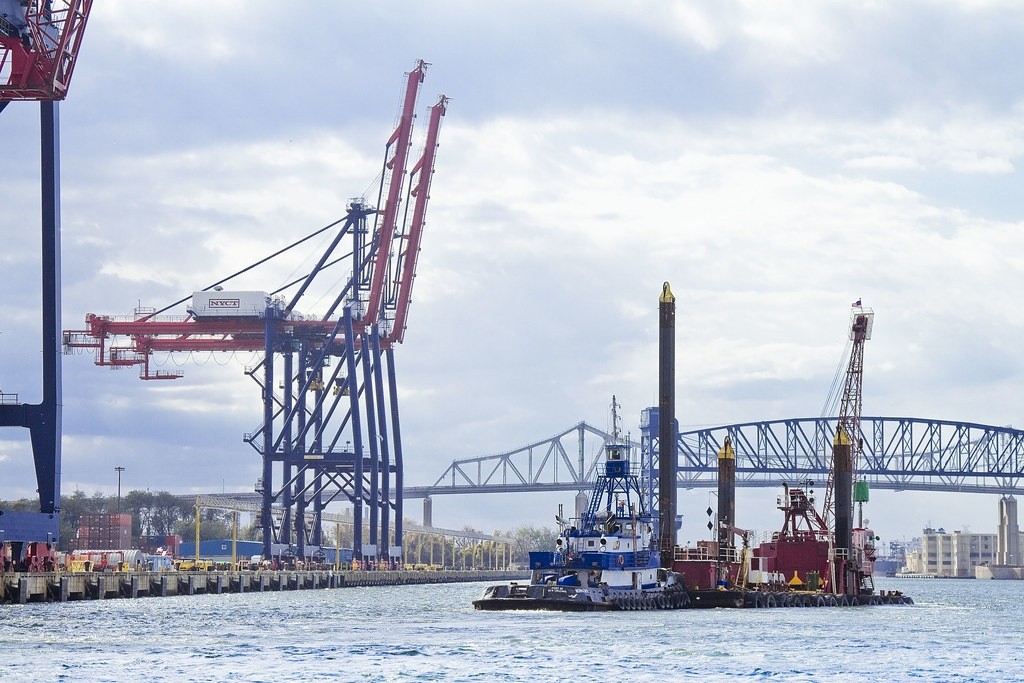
730;574;736;582
618;555;625;564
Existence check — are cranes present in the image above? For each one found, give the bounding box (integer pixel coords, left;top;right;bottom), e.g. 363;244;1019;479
63;55;452;573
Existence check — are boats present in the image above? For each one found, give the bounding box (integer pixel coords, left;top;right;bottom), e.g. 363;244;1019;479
471;279;920;612
